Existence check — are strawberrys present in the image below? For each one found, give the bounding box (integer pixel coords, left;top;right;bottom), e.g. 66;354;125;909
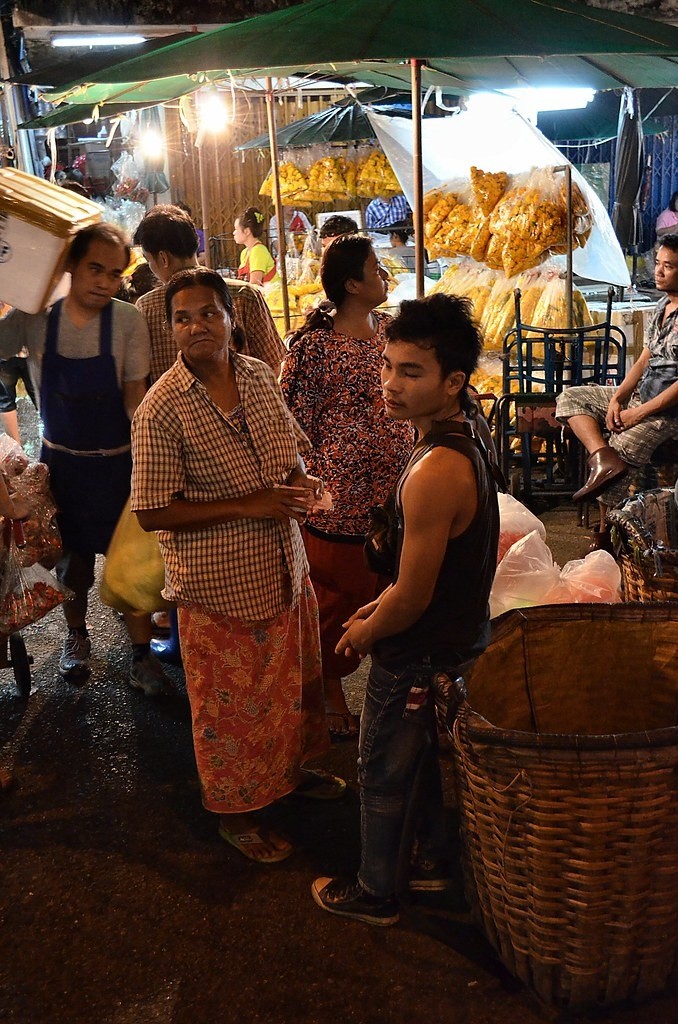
0;581;65;639
22;520;61;567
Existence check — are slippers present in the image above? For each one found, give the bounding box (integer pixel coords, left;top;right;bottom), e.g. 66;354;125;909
282;766;346;798
327;710;358;739
218;822;295;863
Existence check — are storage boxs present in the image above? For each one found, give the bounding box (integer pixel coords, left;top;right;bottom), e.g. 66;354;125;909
590;308;655;382
0;167;108;314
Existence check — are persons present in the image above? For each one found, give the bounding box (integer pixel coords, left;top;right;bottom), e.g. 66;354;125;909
173;201;206;265
231;206;281;289
130;203;290;391
130;266;347;865
269;204;312;258
280;234;415;741
386;230;431;277
655;189;678;240
308;294;499;926
1;188;178;701
553;233;678;518
363;192;411;235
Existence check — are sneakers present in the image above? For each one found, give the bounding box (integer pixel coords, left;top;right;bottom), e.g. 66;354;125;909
59;629;91;677
312;876;400;927
406;853;453;891
130;658;179;698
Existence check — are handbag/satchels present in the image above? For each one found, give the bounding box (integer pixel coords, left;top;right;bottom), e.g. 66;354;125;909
98;492;175;612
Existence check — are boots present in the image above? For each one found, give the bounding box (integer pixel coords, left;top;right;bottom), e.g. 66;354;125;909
583;522;613;557
571;444;628;504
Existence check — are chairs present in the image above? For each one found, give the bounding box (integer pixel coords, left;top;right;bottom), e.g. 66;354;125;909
494;392;590;526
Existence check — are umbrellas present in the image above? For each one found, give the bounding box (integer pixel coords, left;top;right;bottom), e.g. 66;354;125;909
234;90;443;154
0;0;678;300
136;107;170;206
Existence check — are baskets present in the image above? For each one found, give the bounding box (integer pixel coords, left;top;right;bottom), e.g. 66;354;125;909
463;600;676;1009
616;487;678;601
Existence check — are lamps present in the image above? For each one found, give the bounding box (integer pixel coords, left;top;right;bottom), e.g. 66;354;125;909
51;32;146;46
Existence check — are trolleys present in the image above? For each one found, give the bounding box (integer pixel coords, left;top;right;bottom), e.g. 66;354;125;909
1;517;37;697
464;285;629;529
